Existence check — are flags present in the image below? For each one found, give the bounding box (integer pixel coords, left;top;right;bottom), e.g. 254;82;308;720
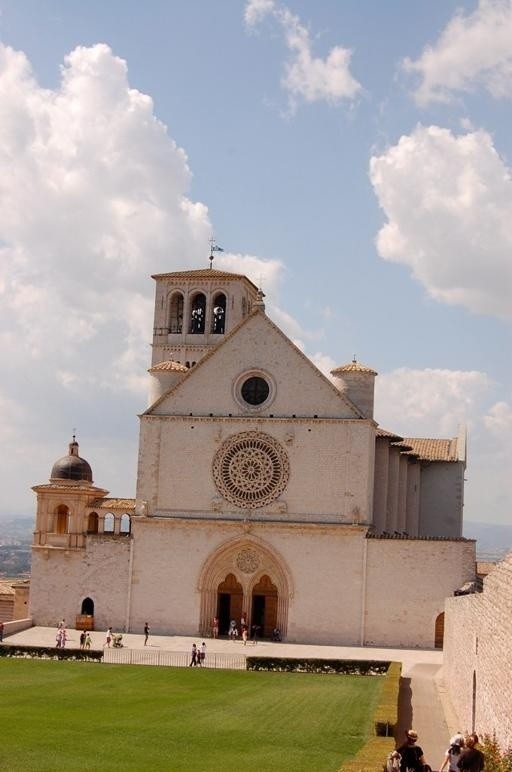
212;243;224;251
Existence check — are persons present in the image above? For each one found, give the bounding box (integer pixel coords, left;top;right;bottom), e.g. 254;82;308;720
198;642;207;667
0;622;3;643
196;650;201;664
440;735;467;772
397;729;426;772
54;618;150;662
456;735;485;772
212;617;282;647
189;644;197;667
